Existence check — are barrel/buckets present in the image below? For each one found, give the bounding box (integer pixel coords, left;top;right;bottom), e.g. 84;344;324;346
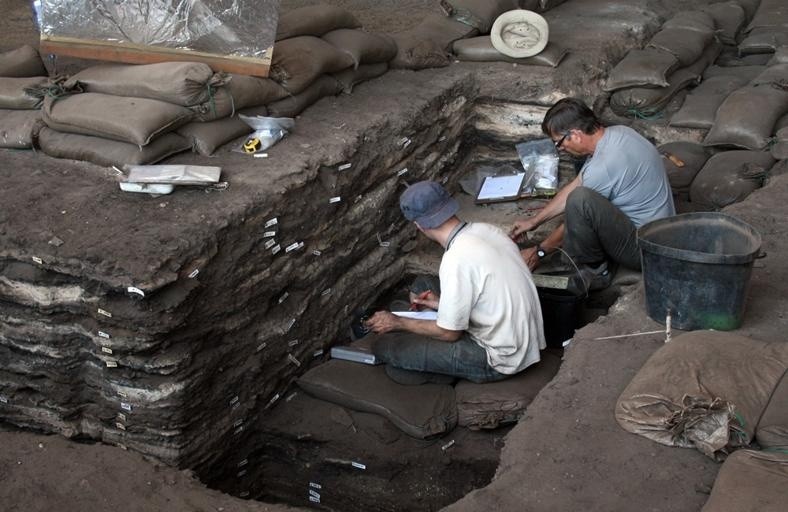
636;213;765;332
528;246;588;350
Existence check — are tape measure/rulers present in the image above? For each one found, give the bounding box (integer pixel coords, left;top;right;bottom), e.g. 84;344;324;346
240;137;262;154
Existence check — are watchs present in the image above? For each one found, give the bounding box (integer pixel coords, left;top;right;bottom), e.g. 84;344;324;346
537;244;547;258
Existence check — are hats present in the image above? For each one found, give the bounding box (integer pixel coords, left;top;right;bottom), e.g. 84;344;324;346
490;10;549;59
399;181;460;228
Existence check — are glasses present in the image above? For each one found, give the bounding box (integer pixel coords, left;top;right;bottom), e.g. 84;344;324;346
555;132;571;153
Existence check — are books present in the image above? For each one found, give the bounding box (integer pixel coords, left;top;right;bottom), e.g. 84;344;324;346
391;311;439;321
477;173;524;199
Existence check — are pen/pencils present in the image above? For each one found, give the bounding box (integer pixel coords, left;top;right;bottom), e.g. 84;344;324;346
408;288;432;311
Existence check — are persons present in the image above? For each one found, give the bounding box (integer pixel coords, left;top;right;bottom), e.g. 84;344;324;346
364;182;547;387
510;98;675;292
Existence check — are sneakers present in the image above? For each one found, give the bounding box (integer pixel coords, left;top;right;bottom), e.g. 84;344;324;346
544;256;613;304
384;365;454;387
